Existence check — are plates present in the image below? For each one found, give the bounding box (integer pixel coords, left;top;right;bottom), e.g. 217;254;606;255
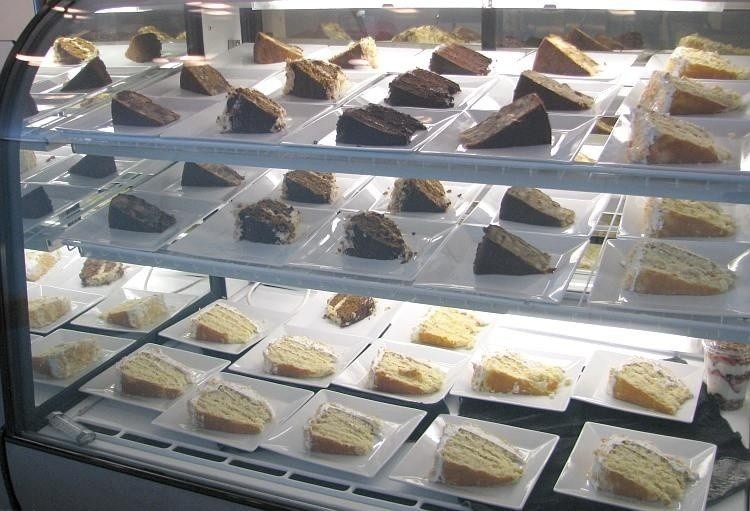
210;42;327;69
330;337;470;403
30;333;43;344
410;223;590;304
310;45;422;72
56;100;219;136
281;105;461;152
137;71;281;100
388;414;560;509
88;45;185;74
151;370;316;452
613;79;750;118
554;422;717;510
464;184;613;235
21;183;97;233
30;67;72;91
291;290;406;346
420;109;598;164
588;237;749;319
26;280;106;334
574;346;705;422
342;72;499;110
19;145;73;182
641;52;749;79
286;211;457;283
31;66;159;98
448;345;585;412
39;254;144;296
617;195;749;241
134;161;272;203
342;175;484;223
24;153;144;191
41;44;104;67
160;100;333;143
232;168;373;210
167;201;338;269
20;92;86;121
160;298;291;355
251;69;386;104
78;342;232;414
229;328;369;390
71;287;196;333
502;48;644;79
258;387;428;479
389;44;526;74
31;329;134;388
60;188;227;252
597;115;750;173
468;75;624;117
380;300;500;356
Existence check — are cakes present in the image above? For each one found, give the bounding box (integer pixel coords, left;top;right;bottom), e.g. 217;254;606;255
253;31;304;63
624;237;736;295
303;400;384;455
473;224;556;274
429;40;492;75
470;347;565;396
676;32;746;54
31;336;97;379
513;69;595;110
281;169;336;202
100;292;170;328
284;57;348;99
564;24;643;52
52;35;99;63
414;306;478;348
79;258;124;287
607;357;694;415
187;377;275;433
120;349;192;397
180;61;234;95
389;24;448;47
238;197;297;245
639;70;740;115
61;55;112;90
428;423;525;486
336;102;426;145
111;89;179;126
384;66;460;108
125;32;163;62
367;350;443;394
328;35;378;68
533;33;603;76
263;334;337;377
387;177;451;212
499;186;575;227
181;161;245;186
623;108;732;164
665;44;749;78
461;92;551;148
216;87;286;133
326;292;375;327
589;434;698;508
310;22;347;42
640;196;736;237
20;149;37;172
68;154;117;178
28;295;72;328
191;302;260;343
338;210;413;264
